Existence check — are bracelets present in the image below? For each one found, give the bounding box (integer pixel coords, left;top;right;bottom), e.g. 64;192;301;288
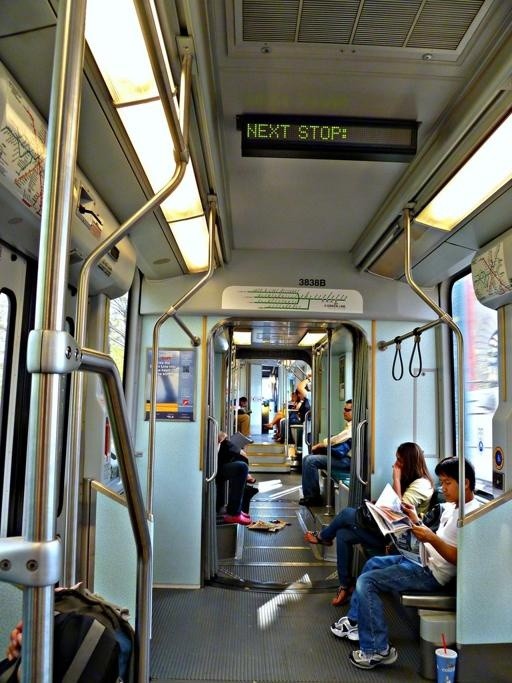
416;519;423;526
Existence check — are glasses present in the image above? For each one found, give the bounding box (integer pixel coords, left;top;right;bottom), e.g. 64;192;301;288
344;408;352;412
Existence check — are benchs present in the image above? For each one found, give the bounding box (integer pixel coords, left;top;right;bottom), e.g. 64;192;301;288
320;466;494;608
289;424;303;446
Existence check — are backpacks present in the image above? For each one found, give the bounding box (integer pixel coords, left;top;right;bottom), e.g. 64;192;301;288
0;583;135;683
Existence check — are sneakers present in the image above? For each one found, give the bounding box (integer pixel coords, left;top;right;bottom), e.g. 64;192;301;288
299;497;322;506
264;423;273;430
331;617;359;640
349;644;398;669
224;511;250;524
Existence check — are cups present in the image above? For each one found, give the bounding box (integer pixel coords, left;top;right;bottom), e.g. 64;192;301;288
435;648;459;682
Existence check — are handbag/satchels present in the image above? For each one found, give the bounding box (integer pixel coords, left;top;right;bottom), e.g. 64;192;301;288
322;438;352;457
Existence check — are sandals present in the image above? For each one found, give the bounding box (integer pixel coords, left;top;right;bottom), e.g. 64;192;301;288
307;531;333;546
336;585;353;599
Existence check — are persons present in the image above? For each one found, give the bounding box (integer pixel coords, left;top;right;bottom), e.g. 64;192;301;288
7;621;21;683
330;455;483;671
263;376;311;445
299;399;353;505
234;397;252;437
226;438;257;483
304;441;436;606
218;425;253;525
